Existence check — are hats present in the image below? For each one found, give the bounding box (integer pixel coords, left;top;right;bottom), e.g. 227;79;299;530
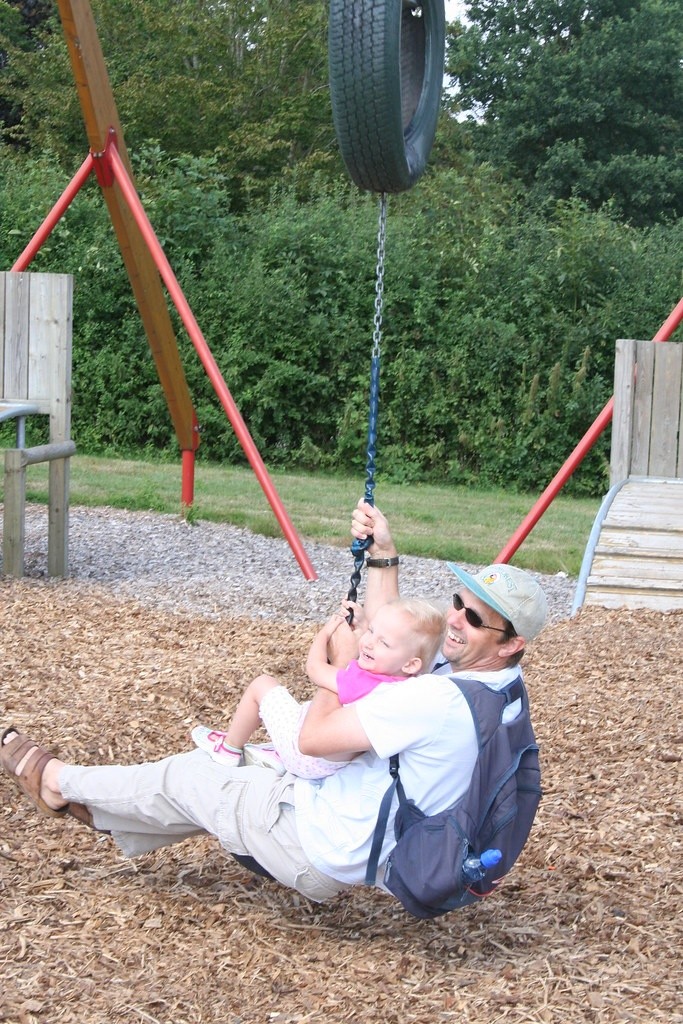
446;560;548;642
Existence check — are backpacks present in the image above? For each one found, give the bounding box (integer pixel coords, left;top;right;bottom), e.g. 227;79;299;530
382;675;542;919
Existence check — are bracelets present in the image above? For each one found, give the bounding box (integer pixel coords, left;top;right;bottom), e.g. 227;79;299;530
366;556;399;567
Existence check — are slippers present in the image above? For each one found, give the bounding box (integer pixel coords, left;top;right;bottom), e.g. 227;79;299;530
0;726;68;816
66;796;112;836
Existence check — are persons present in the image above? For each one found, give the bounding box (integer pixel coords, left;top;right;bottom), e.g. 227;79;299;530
0;561;549;918
190;599;446;780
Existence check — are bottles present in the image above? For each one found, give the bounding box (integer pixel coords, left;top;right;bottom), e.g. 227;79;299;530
460;849;502;887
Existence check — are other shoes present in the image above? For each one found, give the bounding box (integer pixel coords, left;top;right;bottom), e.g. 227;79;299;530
245;740;286;777
191;725;241;766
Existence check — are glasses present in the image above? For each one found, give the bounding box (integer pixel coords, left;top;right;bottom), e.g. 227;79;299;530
452;592;512;635
